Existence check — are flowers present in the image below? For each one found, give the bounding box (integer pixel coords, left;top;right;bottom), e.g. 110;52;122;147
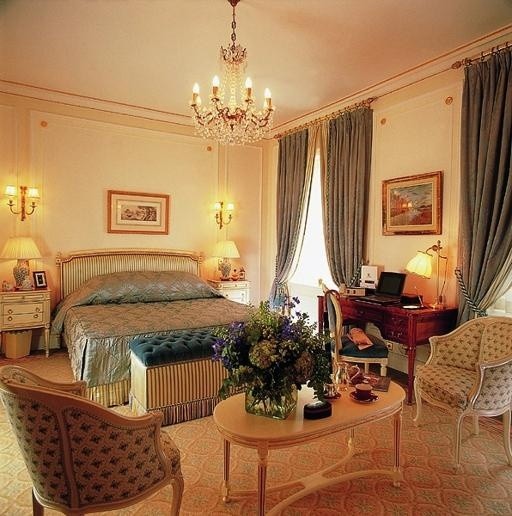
211;295;335;401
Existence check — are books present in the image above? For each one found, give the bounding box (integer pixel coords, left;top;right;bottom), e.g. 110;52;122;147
371;376;392;392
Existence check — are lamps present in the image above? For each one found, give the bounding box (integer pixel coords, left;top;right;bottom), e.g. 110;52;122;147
4;184;40;220
0;236;42;289
190;1;274;146
214;201;234;230
404;239;449;310
212;241;242;279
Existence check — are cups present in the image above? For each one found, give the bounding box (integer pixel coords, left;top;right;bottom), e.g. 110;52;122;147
353;383;373;400
323;383;337;398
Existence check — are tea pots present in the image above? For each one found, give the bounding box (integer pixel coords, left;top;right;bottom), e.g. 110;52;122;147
333;361;361;393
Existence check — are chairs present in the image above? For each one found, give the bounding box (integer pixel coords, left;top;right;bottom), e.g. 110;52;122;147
1;365;185;516
323;280;388;378
412;315;512;475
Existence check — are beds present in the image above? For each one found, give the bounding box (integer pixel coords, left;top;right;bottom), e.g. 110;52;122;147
51;249;288;427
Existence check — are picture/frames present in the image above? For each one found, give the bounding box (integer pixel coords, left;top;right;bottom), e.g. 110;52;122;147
382;170;442;236
32;271;47;288
107;190;170;234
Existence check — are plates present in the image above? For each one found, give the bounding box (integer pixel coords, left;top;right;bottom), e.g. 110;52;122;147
348;392;380;405
319;393;343;402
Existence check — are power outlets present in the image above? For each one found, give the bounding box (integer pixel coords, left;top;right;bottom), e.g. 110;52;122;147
386;342;393;350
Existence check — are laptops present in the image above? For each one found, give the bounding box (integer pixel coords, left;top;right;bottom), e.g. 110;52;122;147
359;272;406;303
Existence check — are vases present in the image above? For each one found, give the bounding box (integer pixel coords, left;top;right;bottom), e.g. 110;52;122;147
243;379;297;418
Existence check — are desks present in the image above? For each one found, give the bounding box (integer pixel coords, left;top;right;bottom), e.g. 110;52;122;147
318;296;458;406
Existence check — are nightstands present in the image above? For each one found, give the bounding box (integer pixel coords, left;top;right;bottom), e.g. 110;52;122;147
0;290;51;360
209;279;249;305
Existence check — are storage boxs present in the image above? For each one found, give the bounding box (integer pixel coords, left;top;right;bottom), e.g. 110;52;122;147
0;330;32;359
